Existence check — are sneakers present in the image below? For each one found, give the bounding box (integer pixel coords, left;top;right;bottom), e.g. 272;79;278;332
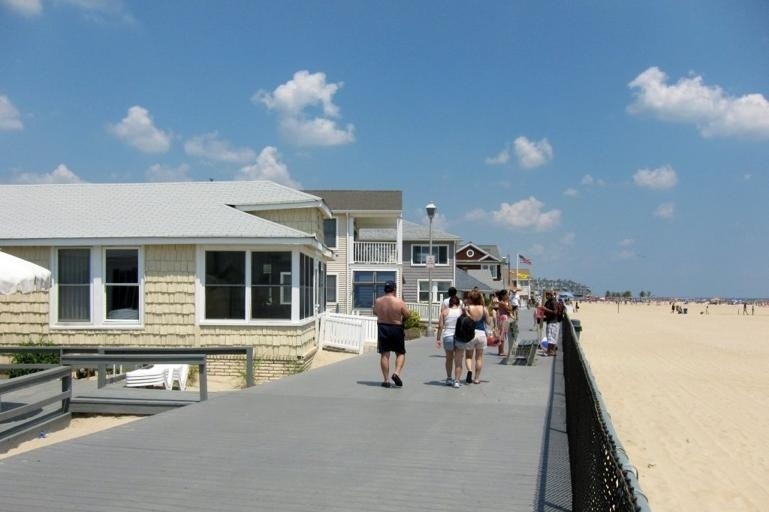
446;377;461;388
382;374;402;387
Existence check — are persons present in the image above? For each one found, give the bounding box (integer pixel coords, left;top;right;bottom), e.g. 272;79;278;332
530;288;568;356
374;280;410;388
743;303;748;315
436;286;491;389
671;303;688;314
489;286;521;356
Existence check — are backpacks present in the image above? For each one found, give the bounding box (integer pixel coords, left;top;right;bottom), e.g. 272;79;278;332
455;309;475;343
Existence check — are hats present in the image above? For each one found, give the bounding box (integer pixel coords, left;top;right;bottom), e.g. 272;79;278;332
385;280;394;287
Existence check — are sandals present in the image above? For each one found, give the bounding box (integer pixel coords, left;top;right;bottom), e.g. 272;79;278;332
466;371;481;384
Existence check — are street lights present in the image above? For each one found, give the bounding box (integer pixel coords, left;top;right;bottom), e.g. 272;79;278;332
425;200;438;337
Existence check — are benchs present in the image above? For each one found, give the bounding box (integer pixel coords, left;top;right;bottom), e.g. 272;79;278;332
512;338;540;366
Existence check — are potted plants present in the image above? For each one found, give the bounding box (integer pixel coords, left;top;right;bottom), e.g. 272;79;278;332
403;309;420;340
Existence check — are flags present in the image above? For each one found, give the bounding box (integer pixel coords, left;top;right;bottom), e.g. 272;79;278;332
519;255;532;266
